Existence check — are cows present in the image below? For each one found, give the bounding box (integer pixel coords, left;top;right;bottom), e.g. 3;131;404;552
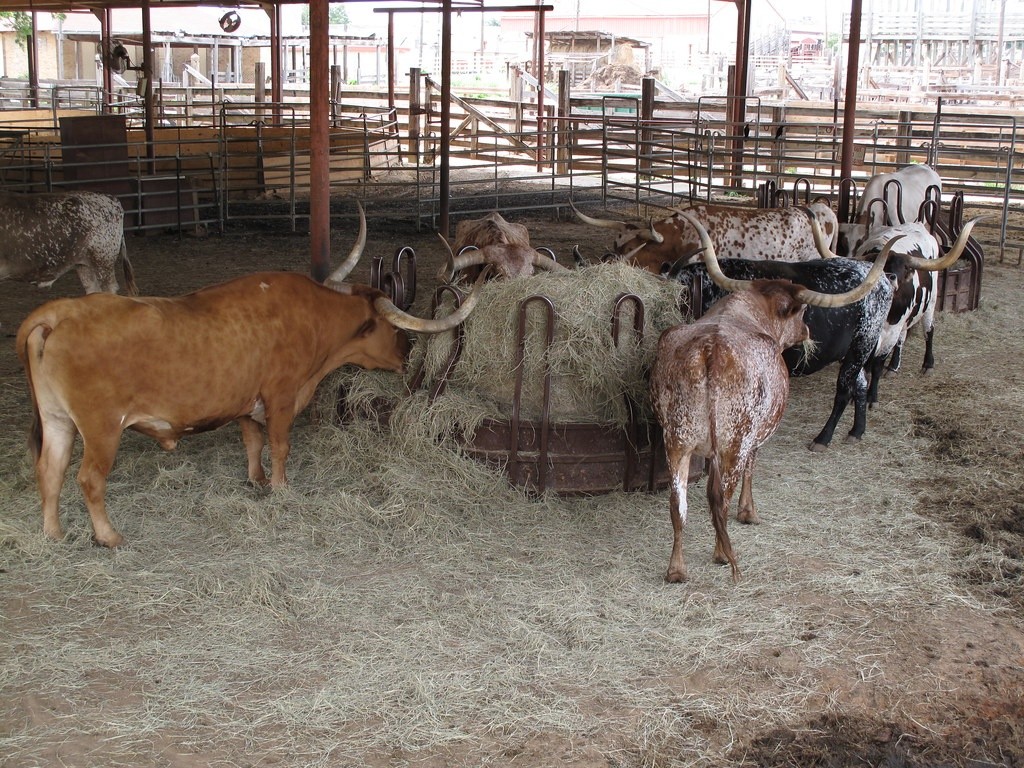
15;199;493;550
0;191;139;307
657;258;895;453
646;205;907;583
434;211;573;286
568;196;839;277
836;161;999;412
